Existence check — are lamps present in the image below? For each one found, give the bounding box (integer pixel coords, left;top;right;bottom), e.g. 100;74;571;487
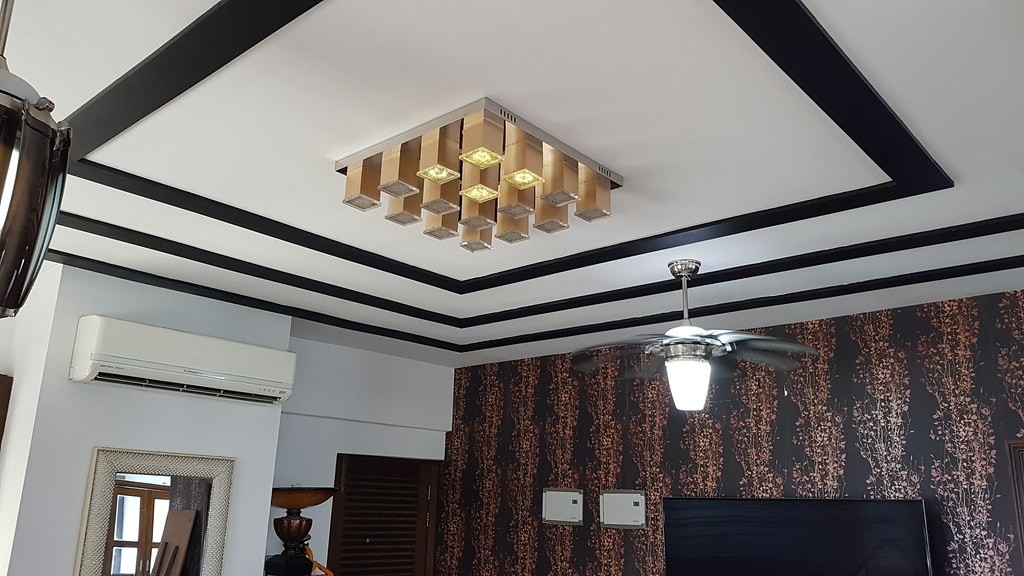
264;484;339;575
661;342;714;412
336;98;622;259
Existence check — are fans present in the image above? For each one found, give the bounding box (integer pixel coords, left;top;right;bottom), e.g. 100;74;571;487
566;259;822;380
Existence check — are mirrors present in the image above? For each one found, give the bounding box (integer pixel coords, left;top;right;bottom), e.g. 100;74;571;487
74;446;236;575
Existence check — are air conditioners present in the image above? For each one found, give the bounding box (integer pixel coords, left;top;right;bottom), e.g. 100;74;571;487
68;314;298;405
